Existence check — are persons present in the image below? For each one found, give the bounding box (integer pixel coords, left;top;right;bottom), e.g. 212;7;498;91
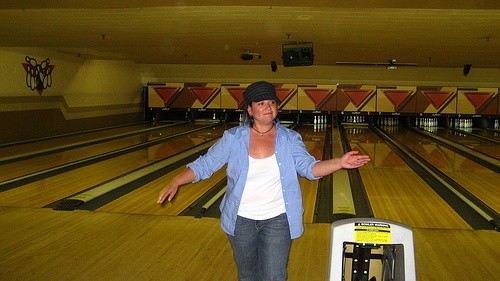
157;81;371;281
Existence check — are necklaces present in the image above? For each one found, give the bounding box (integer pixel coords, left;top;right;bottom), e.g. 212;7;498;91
250;123;275;137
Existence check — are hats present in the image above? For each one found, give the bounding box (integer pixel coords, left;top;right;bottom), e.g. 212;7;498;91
243;80;278;102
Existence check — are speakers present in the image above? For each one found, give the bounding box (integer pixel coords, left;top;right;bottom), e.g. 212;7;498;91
283;47;313;67
